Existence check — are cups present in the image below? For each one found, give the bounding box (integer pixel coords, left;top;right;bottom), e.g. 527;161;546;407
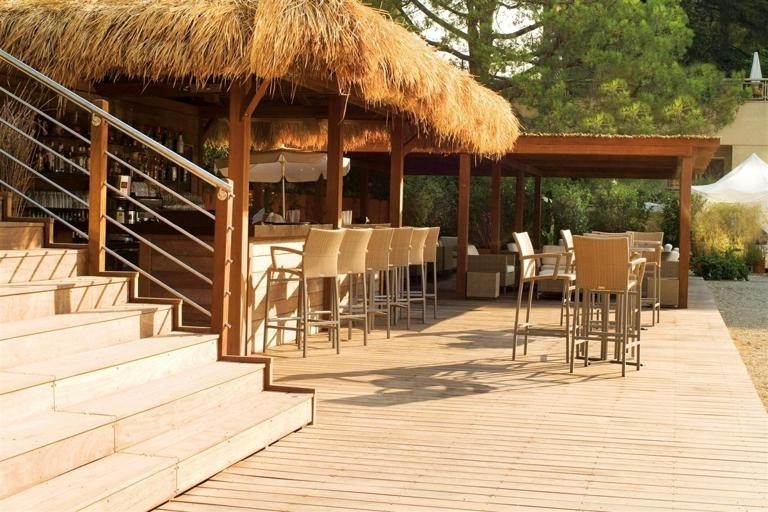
342;210;353;226
286;209;301;224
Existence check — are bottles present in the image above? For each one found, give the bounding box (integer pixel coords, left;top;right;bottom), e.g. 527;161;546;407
30;110;191;183
114;205;137;225
25;189;74;209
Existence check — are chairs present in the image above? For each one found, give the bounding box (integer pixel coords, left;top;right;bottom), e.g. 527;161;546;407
467;229;680;377
261;225;443;358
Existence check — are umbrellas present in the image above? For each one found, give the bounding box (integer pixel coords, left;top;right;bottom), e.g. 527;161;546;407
212;141;351;219
749;51;763;83
690;150;768;235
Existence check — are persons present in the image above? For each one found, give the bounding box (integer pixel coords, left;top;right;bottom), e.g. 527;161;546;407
251;198;286;225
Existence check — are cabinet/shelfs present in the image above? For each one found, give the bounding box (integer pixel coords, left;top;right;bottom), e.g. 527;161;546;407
4;80;200;247
